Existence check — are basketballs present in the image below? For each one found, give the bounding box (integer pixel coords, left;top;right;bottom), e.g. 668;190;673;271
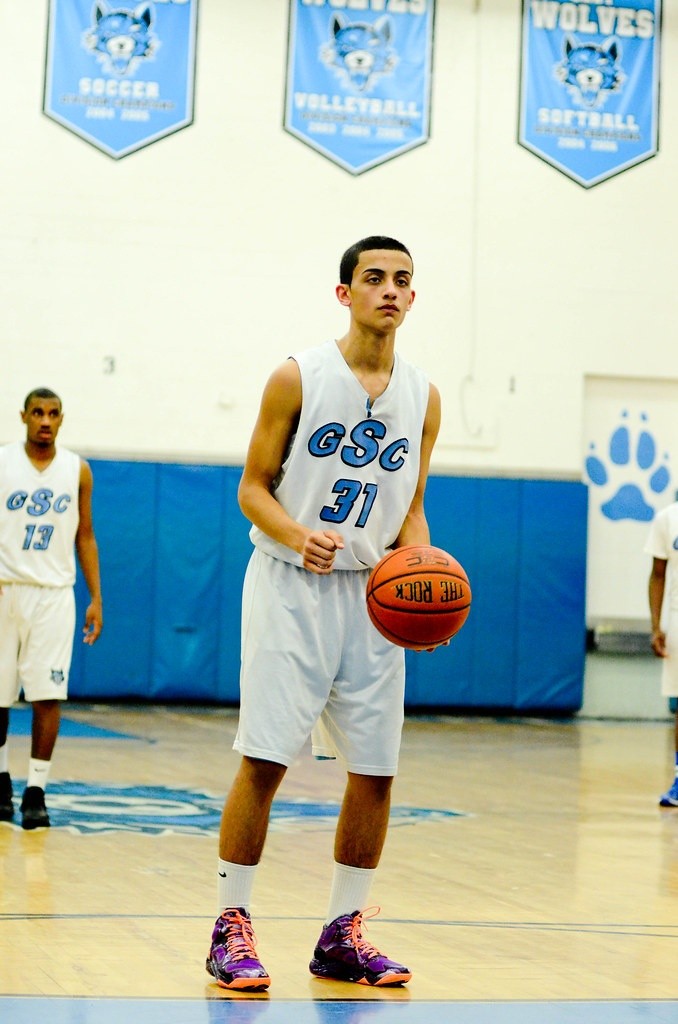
366;545;471;651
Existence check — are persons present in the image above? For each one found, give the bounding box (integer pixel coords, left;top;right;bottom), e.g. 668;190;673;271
0;388;101;830
645;502;678;807
205;235;450;991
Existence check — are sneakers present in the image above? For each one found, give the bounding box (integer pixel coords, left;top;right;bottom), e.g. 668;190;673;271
20;786;51;830
0;771;14;821
205;907;271;992
309;905;412;987
659;776;678;807
